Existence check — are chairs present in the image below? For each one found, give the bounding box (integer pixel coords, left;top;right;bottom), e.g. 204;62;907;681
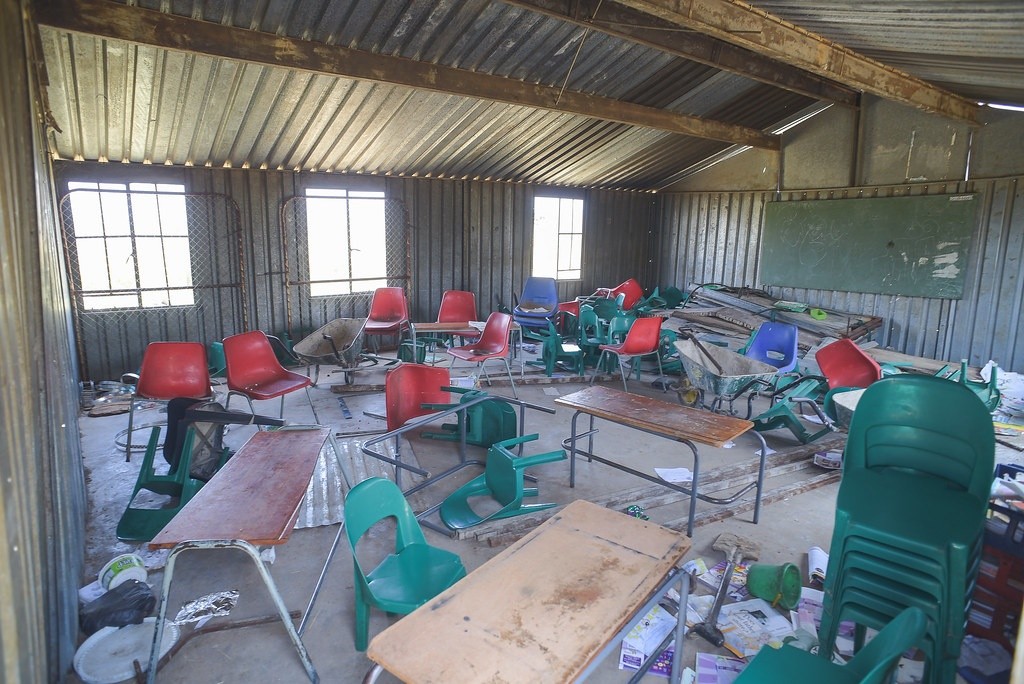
113;276;1024;684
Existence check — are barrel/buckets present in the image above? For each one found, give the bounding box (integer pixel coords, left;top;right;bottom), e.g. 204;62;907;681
97;554;148;591
746;562;802;611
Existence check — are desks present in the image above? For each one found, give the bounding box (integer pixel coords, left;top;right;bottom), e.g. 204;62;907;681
365;499;693;684
410;321;524;379
555;386;767;538
147;427;359;684
